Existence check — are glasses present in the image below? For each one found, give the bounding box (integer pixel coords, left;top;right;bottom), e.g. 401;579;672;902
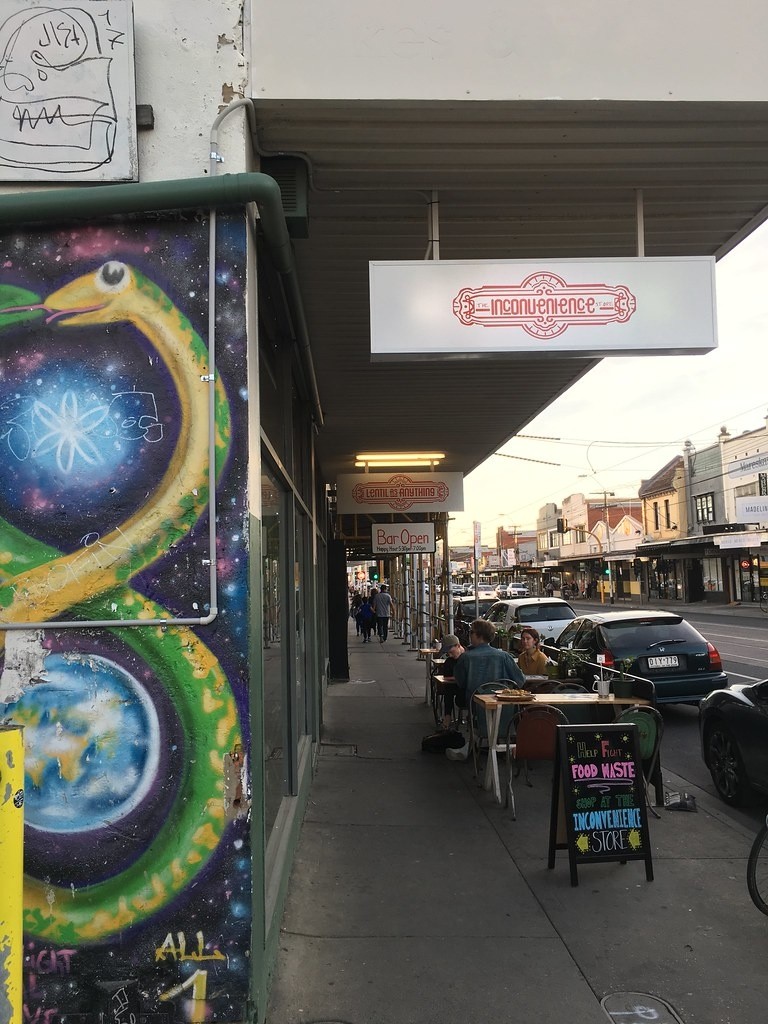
469;630;479;635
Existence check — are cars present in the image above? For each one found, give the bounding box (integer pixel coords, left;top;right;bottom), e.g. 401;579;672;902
696;678;768;807
419;582;530;599
436;597;500;647
469;597;579;652
367;581;390;592
660;579;683;590
542;608;728;713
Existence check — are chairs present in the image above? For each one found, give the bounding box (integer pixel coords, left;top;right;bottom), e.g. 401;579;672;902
549;683;600;727
611;705;666;819
530;679;562;696
468;682;532;788
504;705;570;821
493;679;523;692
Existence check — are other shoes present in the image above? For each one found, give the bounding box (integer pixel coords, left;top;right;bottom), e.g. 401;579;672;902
356;630;387;644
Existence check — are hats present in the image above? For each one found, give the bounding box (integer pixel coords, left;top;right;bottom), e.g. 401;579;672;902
441;634;459;653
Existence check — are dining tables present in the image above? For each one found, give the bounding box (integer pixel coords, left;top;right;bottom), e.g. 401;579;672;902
420;647;441;706
430;659;559;722
431;674;583;732
473;691;651;803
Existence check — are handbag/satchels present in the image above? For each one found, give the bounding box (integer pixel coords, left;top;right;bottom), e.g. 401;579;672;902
421;727;464;752
444;741;471;760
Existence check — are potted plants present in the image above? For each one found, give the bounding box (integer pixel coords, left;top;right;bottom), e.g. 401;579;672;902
560;648;582;677
609;655;635;698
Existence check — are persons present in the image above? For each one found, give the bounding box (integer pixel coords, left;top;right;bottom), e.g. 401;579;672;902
350;594;367;637
454;617;526;749
368;587;381;637
355;596;375;643
372;584;396;644
545;580;555;600
517;627;551;676
438;634;474;739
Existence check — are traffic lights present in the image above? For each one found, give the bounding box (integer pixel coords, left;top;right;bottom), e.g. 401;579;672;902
594;566;602;574
602;567;610;574
368;566;378;579
355;572;365;579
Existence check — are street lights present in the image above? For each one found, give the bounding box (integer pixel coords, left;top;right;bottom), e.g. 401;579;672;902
578;474;616;604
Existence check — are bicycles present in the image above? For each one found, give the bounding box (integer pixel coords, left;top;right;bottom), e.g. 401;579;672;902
746;814;768;915
760;592;768;614
